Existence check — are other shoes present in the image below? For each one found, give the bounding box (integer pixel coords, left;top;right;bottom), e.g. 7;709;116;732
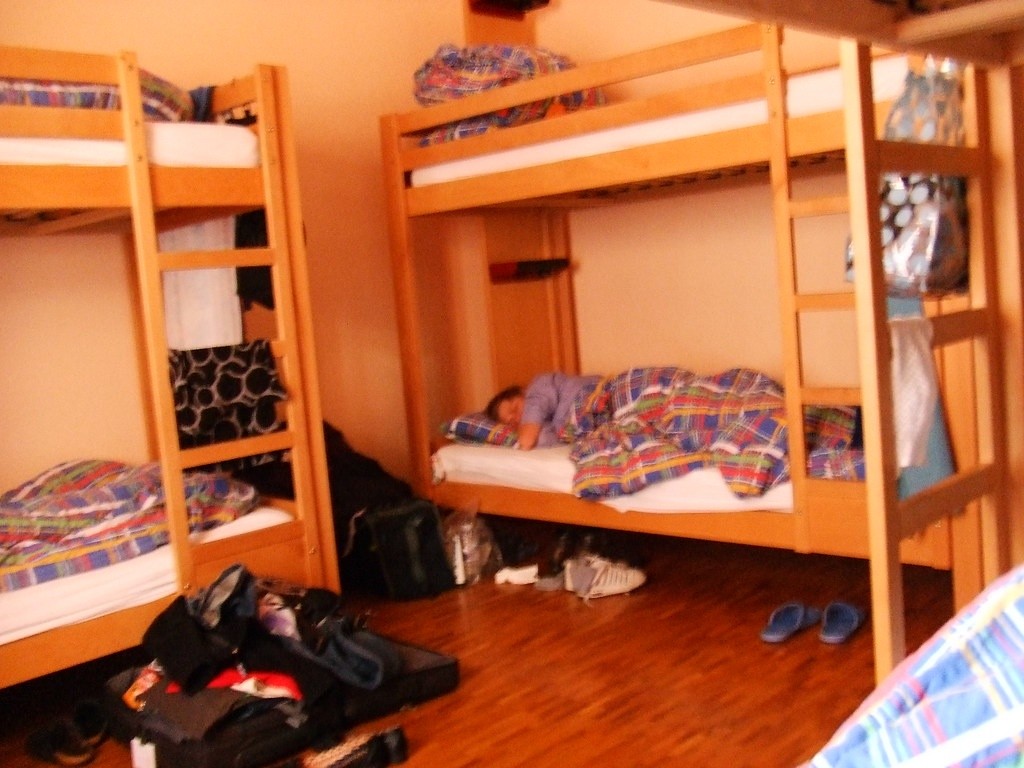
548;531;605;575
26;696;110;764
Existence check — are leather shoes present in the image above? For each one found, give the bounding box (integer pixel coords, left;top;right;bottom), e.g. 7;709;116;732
290;723;410;768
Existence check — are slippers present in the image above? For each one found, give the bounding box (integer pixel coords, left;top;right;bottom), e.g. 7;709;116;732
761;596;864;644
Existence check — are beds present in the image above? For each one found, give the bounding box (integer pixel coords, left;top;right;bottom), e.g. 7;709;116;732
660;0;1024;768
0;46;340;689
377;13;986;613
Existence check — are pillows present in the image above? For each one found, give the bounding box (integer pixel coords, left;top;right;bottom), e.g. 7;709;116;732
439;412;518;448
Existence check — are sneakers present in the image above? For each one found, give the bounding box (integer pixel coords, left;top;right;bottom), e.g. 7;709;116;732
563;558;648;599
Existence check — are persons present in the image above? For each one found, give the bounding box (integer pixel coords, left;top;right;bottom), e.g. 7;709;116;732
484;369;602;452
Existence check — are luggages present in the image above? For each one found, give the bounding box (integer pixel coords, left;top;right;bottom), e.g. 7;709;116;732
103;620;465;768
